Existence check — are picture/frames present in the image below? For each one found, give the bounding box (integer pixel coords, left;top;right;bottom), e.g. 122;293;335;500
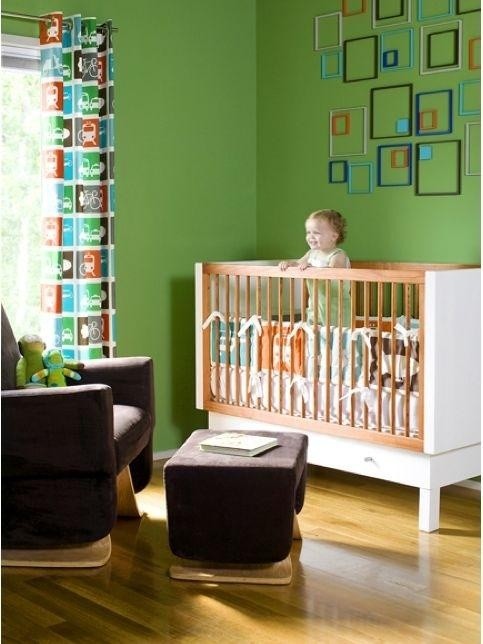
313;0;480;197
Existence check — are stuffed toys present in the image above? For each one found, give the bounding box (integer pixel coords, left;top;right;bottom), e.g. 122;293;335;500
31;348;82;387
15;334;84;389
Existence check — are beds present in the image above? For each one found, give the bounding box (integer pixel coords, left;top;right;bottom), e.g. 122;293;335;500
194;256;480;535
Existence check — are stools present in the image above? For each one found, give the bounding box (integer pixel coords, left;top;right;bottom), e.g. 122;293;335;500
164;427;309;570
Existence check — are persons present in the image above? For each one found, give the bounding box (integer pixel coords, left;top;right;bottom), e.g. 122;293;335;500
278;208;352;329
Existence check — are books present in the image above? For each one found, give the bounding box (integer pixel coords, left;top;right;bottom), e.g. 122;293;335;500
199;431;278;457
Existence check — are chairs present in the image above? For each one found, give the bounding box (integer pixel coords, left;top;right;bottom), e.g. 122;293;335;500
1;297;157;552
